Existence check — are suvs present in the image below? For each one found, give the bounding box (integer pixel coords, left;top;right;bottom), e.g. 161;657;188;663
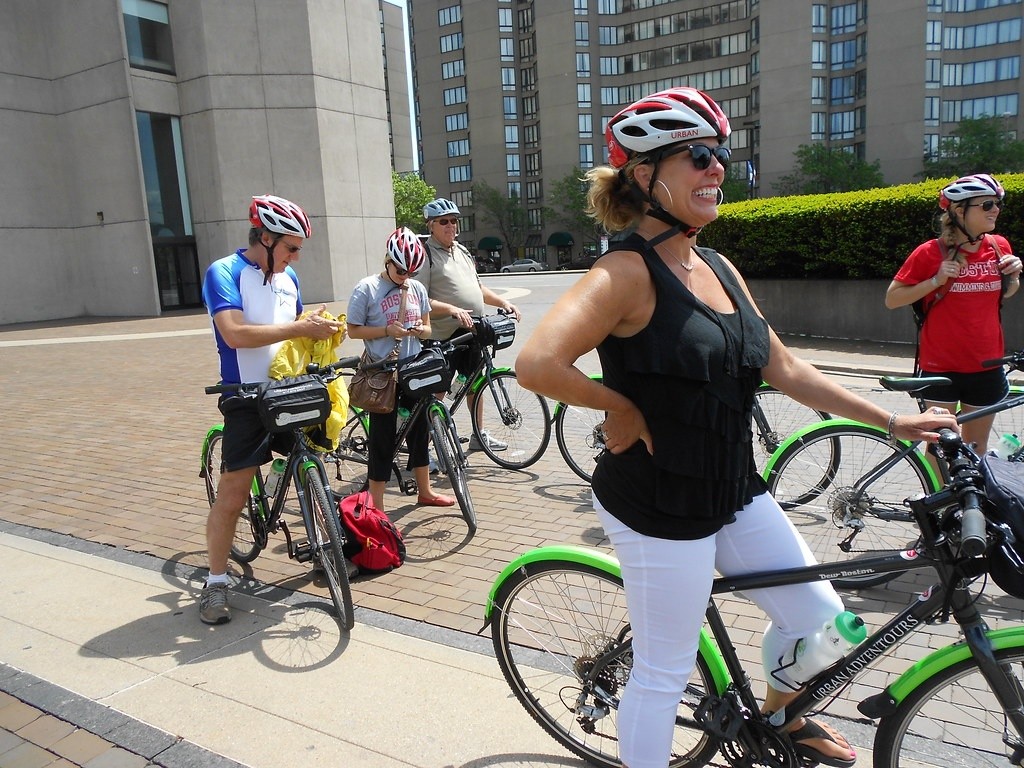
472;255;497;273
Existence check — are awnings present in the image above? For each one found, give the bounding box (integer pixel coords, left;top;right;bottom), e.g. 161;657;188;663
548;231;575;246
478;236;503;250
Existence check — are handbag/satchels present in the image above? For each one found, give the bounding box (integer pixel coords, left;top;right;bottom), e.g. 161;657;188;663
978;451;1024;600
254;374;332;435
479;315;516;350
347;339;452;413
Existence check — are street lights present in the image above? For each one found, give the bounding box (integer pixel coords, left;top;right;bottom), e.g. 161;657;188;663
751;124;761;200
1003;112;1010;143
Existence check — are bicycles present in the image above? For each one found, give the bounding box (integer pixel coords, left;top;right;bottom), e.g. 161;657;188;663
308;307;551;532
473;421;1024;768
552;375;842;509
763;351;1023;600
199;357;360;632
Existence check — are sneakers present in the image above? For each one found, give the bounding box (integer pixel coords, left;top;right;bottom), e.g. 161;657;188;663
199;582;233;625
429;456;439;474
313;549;358;580
469;430;508;451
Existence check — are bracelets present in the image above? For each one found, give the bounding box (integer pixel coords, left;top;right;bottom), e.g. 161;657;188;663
385;324;389;337
1009;277;1019;283
886;411;900;444
931;275;940;289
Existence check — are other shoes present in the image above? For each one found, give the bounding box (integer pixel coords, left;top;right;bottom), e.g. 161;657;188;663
418;493;455;506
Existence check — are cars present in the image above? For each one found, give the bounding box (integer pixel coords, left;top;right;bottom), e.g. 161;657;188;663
500;258;549;273
556;255;598;271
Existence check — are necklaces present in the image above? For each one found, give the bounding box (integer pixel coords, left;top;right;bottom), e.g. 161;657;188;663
637;227;695;270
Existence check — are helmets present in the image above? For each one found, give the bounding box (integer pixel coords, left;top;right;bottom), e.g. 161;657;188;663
606;87;732;168
248;194;311;238
386;226;426;273
939;174;1004;212
423;198;460;223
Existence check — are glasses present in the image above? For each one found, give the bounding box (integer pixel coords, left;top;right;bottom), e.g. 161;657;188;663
392;261;419;278
644;143;732;171
281;241;303;253
433;218;458;225
958;200;1001;212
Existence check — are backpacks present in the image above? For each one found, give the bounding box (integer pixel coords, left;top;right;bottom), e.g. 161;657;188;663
336;491;406;572
911;235;1011;378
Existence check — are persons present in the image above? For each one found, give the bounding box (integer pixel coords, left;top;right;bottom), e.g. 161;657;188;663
885;174;1023;488
346;226;455;511
198;193;359;624
515;87;959;768
411;198;522;474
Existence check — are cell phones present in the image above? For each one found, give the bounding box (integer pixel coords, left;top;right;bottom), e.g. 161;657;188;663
406;326;418;331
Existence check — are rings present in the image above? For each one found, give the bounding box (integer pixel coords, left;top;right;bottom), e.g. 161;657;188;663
933;408;942;414
604;431;609;441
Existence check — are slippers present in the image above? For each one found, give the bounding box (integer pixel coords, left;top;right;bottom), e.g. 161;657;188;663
789;715;857;768
